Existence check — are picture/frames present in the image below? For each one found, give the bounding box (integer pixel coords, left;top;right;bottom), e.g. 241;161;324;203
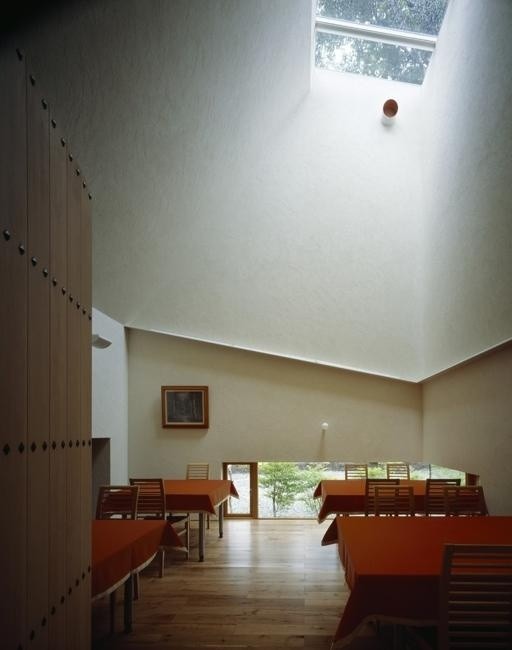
160;385;211;430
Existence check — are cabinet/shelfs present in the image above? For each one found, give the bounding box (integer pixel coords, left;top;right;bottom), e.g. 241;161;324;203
0;15;98;649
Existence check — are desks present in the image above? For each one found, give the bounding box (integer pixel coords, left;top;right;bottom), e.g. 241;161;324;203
321;515;512;649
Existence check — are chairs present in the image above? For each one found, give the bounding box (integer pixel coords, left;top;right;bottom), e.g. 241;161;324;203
313;465;488;518
91;463;236;632
438;544;512;649
